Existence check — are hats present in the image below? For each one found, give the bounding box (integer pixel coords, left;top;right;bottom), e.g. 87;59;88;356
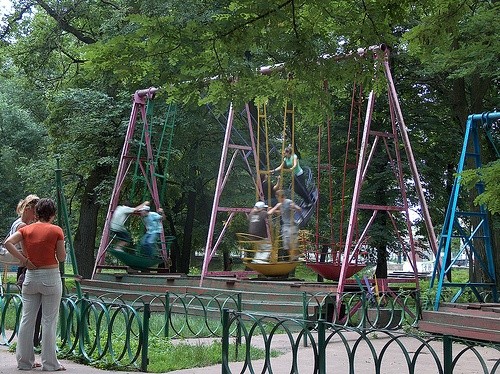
255;202;268;208
138;206;150;211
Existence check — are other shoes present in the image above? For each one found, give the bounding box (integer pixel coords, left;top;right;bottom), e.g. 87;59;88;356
114;248;124;252
54;365;66;371
32;363;42;367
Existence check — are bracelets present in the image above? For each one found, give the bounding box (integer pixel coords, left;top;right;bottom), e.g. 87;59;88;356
23;257;29;265
271;169;276;174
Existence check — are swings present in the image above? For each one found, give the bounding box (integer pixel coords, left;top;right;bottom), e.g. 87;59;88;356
305;59;369;281
107;93;177;271
204;92;319;228
236;71;309;277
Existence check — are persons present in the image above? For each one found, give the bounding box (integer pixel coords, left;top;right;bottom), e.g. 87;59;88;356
109;199;166;259
4;198;65;371
246;143;315;265
10;194;49;352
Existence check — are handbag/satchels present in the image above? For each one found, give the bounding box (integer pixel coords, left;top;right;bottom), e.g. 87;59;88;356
0;221;22;265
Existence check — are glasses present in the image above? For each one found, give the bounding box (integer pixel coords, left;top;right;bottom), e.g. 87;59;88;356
26;199;39;206
